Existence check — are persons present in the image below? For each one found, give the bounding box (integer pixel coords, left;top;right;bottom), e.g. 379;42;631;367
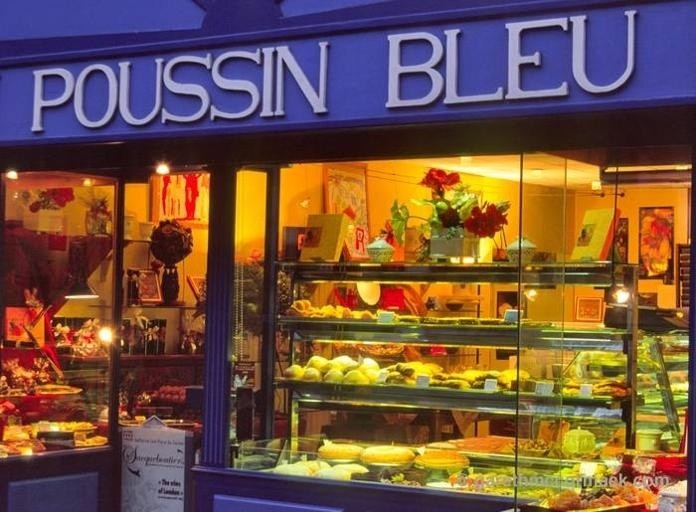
577;228;590;247
305;231;318;247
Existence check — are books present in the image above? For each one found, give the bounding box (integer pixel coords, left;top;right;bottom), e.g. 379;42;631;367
569;206;622;261
299;212;350;262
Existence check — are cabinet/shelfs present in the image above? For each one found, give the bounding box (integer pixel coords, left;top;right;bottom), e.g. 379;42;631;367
232;258;689;508
91;236;206;310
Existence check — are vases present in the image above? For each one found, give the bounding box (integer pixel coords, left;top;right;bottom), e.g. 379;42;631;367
431;223;481;263
490;247;509;264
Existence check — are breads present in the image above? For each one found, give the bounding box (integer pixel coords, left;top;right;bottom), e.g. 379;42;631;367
283;299;630;469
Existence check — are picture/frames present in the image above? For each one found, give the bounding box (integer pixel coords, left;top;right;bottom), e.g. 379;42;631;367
185;275;206;304
138;269;163;305
322;160;370;243
638;206;674;280
608;218;629;263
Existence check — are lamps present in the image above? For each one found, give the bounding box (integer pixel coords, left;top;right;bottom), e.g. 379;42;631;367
64;237;100;300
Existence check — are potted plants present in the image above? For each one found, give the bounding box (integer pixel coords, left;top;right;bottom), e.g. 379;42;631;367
75;186;113;236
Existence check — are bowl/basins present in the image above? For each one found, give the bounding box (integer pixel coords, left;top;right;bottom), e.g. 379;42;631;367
367;248;395;263
507;248;535;264
446;303;463;311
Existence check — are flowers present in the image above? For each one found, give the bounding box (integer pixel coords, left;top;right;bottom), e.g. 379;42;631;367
390;168;509;244
465;202;508;261
29;186;75;212
